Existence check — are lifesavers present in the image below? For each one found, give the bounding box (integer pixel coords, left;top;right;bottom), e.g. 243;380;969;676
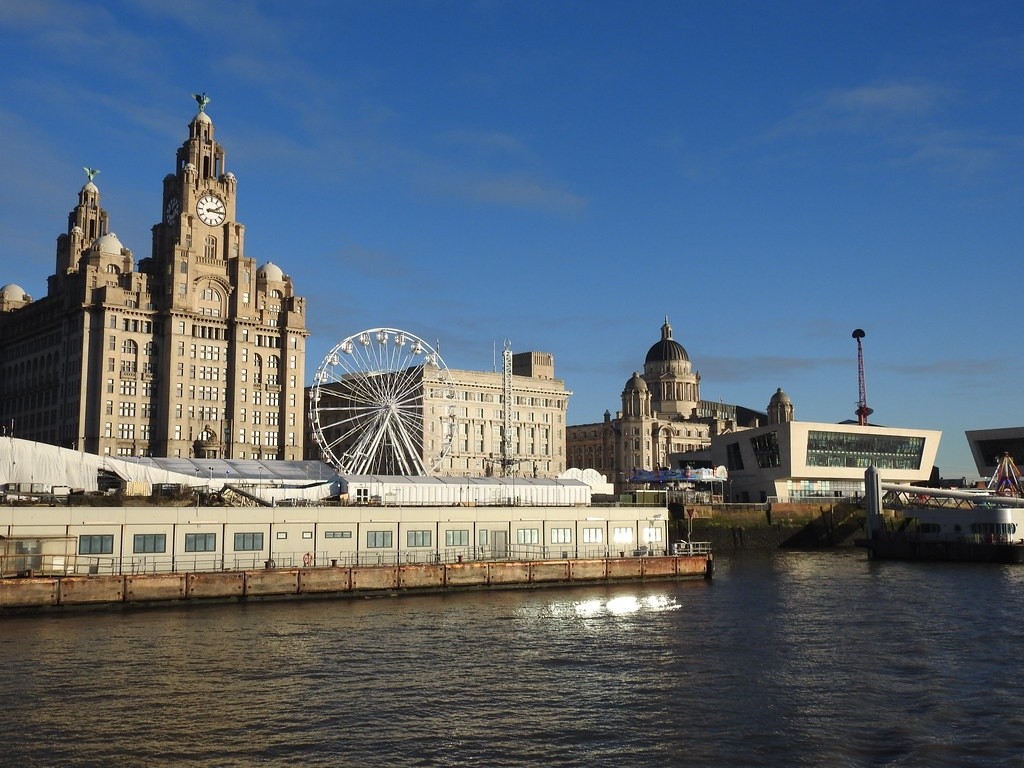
303;553;314;566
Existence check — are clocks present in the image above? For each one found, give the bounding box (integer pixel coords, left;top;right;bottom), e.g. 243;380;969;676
197;195;226;226
